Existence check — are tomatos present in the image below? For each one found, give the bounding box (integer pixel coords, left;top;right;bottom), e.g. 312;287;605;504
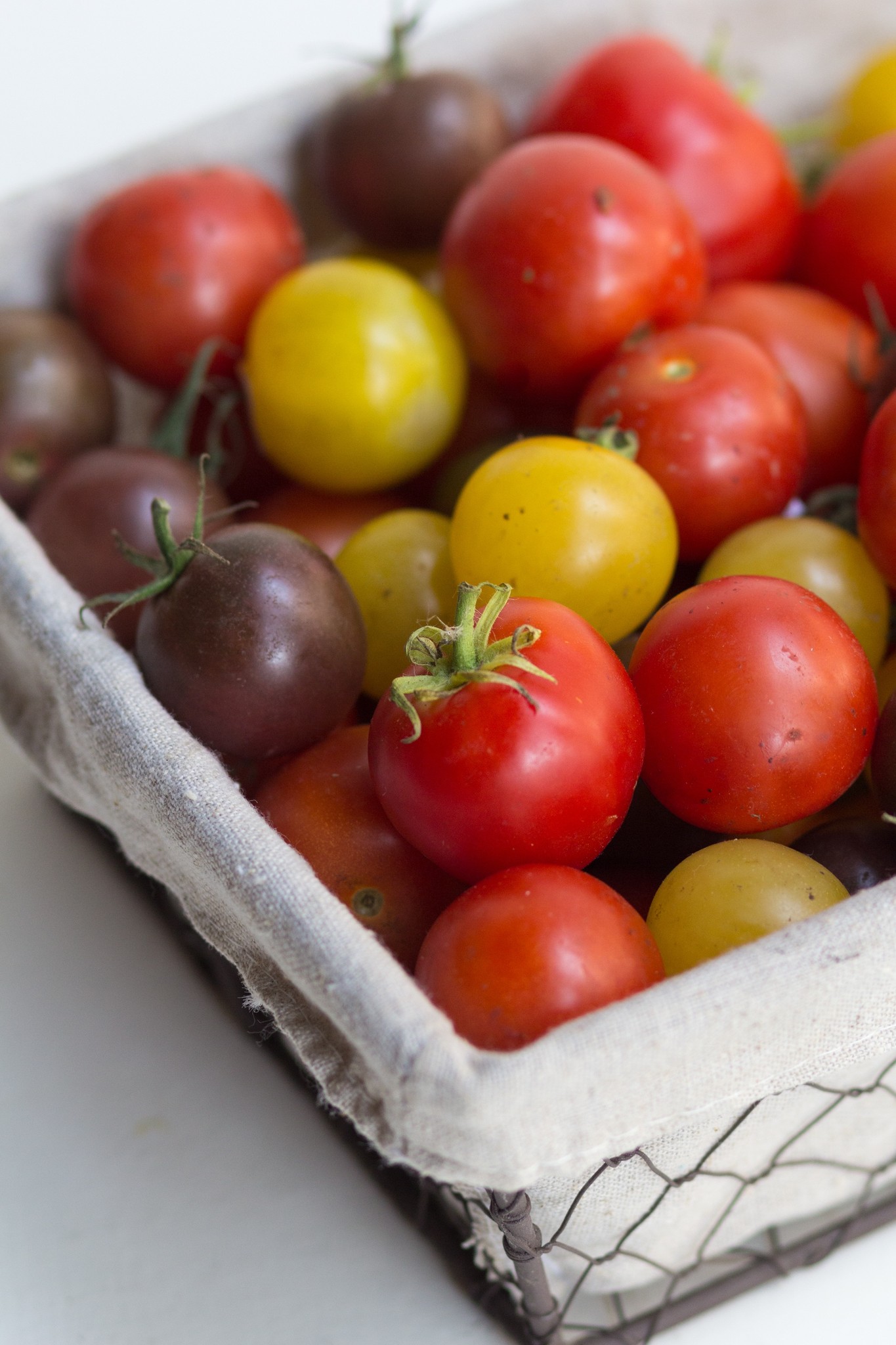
3;7;894;1054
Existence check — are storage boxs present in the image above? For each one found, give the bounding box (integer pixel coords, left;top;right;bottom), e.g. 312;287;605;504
2;0;896;1343
